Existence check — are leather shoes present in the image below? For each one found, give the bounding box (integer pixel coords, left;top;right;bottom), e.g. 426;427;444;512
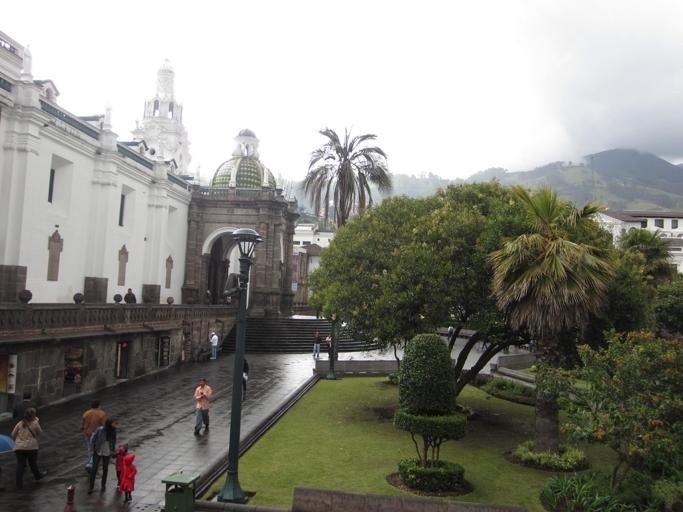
35;470;46;480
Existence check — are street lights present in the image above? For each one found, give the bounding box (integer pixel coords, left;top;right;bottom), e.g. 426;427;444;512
225;227;263;469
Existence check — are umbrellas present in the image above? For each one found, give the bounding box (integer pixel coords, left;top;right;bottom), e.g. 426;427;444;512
0;434;17;455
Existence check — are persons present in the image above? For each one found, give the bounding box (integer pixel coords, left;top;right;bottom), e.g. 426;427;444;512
124;288;135;303
316;308;323;320
72;370;82;393
445;323;454;341
325;333;332;359
312;331;323;357
193;378;211;436
79;400;137;503
242;358;249;402
12;407;48;489
209;332;218;360
